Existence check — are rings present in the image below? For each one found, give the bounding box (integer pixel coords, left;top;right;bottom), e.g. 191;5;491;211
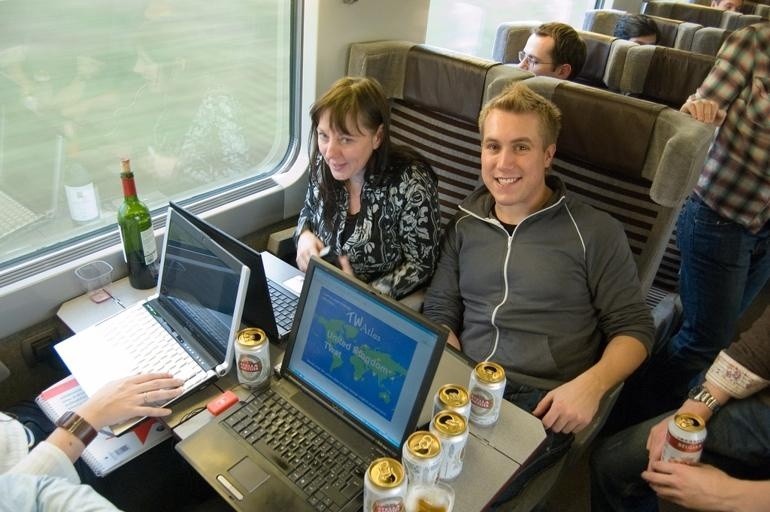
144;391;148;404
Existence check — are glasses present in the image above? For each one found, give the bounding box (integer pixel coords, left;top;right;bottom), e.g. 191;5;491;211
518;51;555;71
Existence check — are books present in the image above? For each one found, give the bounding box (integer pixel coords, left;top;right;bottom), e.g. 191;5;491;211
34;374;173;479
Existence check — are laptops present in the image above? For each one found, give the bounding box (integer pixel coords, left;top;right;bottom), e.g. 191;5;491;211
170;200;309;344
171;255;449;512
52;207;251;437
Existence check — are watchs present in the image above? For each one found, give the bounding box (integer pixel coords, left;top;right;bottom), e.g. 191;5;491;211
687;383;720;412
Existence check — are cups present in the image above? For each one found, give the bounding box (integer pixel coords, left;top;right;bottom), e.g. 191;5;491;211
404;482;455;512
73;262;114;302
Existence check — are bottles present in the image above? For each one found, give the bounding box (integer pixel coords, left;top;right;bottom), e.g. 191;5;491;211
114;159;158;290
61;140;100;226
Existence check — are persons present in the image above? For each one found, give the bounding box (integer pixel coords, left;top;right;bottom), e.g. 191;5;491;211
709;0;744;12
292;76;442;301
588;303;770;512
0;474;125;512
613;13;660;45
0;372;185;484
110;26;259;192
420;81;658;507
517;22;588;81
649;21;770;401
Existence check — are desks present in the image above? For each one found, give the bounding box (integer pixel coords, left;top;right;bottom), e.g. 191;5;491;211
55;276;548;512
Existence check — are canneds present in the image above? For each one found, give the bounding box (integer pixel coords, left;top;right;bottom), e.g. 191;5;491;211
232;326;272;390
357;362;507;512
661;411;707;466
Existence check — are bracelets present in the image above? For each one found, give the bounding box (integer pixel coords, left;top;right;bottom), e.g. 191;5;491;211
57;411;98;448
